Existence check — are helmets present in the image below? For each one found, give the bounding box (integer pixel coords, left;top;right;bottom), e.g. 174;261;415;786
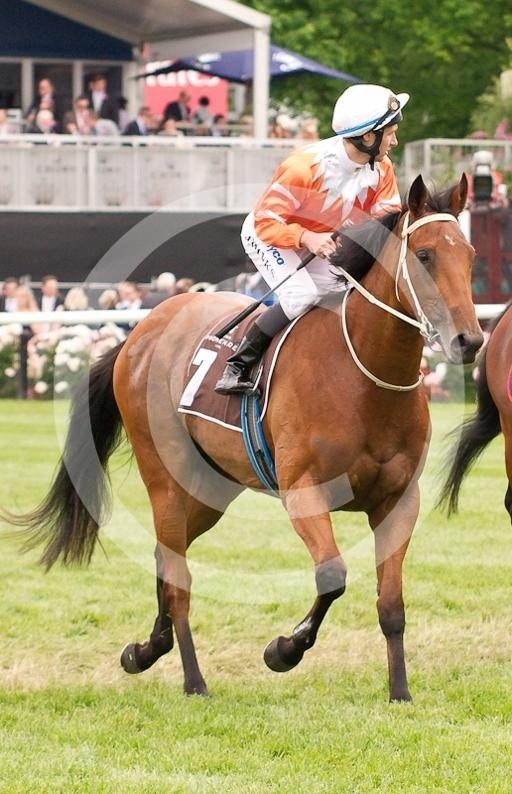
331;84;410;138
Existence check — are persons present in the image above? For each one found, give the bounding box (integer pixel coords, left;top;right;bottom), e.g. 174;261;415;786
209;80;412;395
0;265;276;397
1;69;323;147
462;149;509;208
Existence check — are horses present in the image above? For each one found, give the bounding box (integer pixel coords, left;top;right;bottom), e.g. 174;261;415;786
0;170;486;705
436;300;511;526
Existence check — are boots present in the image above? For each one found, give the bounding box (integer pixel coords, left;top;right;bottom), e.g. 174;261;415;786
215;337;263;396
